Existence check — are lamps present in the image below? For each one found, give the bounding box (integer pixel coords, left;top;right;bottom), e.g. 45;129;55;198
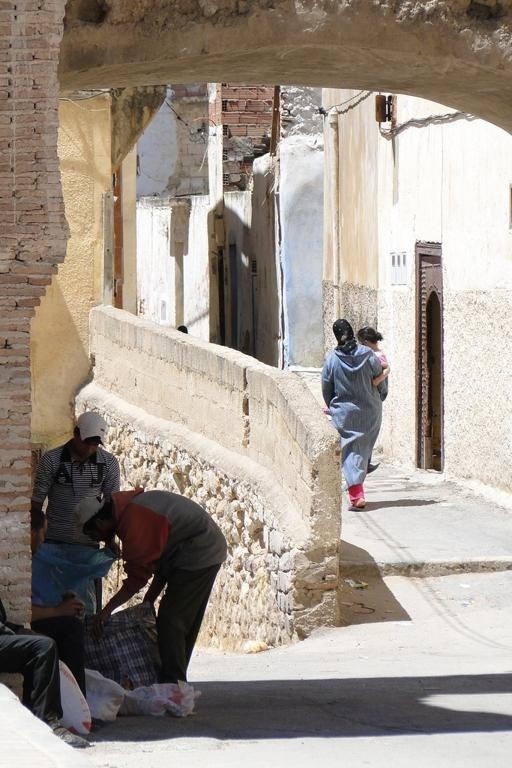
375;91;394;124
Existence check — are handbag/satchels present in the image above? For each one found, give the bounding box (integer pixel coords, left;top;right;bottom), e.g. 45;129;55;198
83;602;161;694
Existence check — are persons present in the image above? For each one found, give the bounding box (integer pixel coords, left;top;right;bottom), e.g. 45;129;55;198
356;326;391;387
321;319;388;512
0;597;89;748
30;412;120;699
72;487;227;685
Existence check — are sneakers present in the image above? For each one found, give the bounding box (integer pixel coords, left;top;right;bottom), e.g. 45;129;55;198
50;720;88;748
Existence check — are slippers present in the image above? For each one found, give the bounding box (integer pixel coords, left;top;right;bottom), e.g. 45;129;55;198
348;503;366;511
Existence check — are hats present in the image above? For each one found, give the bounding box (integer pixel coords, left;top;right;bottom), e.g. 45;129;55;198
74;496;106;539
77;412;107;446
